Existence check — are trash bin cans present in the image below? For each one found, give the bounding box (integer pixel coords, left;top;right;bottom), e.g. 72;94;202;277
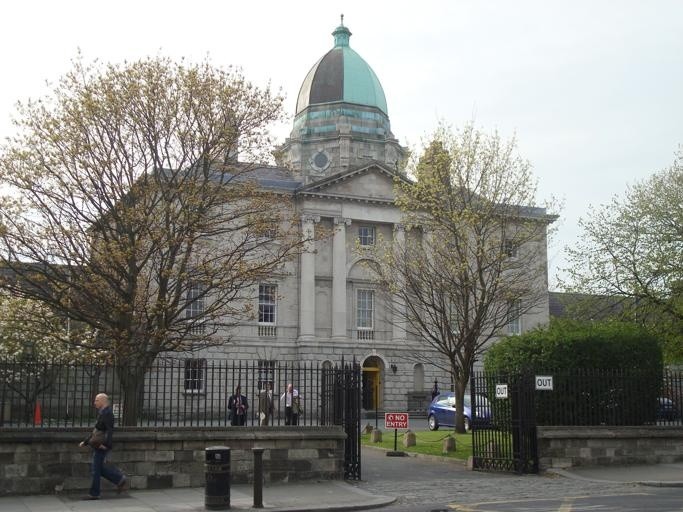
205;446;231;511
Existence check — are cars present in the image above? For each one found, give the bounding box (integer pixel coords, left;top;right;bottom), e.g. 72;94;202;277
426;390;492;432
656;396;676;418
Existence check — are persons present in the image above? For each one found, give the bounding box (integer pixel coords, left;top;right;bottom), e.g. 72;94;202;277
78;393;129;500
257;383;275;426
280;383;301;425
228;386;249;426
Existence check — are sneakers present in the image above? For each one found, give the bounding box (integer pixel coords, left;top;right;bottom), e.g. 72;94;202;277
82;495;100;500
118;481;125;494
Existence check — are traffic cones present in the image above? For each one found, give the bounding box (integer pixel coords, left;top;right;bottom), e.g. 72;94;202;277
34;400;42;425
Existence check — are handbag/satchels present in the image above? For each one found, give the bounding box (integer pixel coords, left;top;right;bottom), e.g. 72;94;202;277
89;430;105;449
